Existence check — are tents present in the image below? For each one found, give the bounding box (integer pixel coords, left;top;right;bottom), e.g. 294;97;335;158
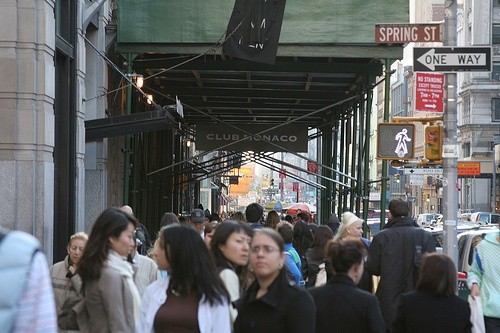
288;203;316;218
264;201;286;211
274;200;294;214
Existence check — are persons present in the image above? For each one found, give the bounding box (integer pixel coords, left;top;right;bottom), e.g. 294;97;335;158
370;198;437;324
308;236;387;333
137;226;232;333
232;228;314;333
0;226;58;333
391;254;473;333
49;203;379;320
466;223;500;333
58;208;141;333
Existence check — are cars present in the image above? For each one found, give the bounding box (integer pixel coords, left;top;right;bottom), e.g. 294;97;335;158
417;211;500;274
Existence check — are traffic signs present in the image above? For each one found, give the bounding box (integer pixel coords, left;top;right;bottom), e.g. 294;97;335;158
413;46;493;73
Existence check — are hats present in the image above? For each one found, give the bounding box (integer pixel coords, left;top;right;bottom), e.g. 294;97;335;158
191;209;205;222
246;203;263;222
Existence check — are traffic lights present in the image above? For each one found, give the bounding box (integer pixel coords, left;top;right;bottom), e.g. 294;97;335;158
424;126;443;161
376;122;415;161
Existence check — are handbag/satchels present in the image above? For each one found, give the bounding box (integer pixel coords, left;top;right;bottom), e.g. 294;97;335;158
468;295;485;333
57;296;84;331
315;263;327;288
